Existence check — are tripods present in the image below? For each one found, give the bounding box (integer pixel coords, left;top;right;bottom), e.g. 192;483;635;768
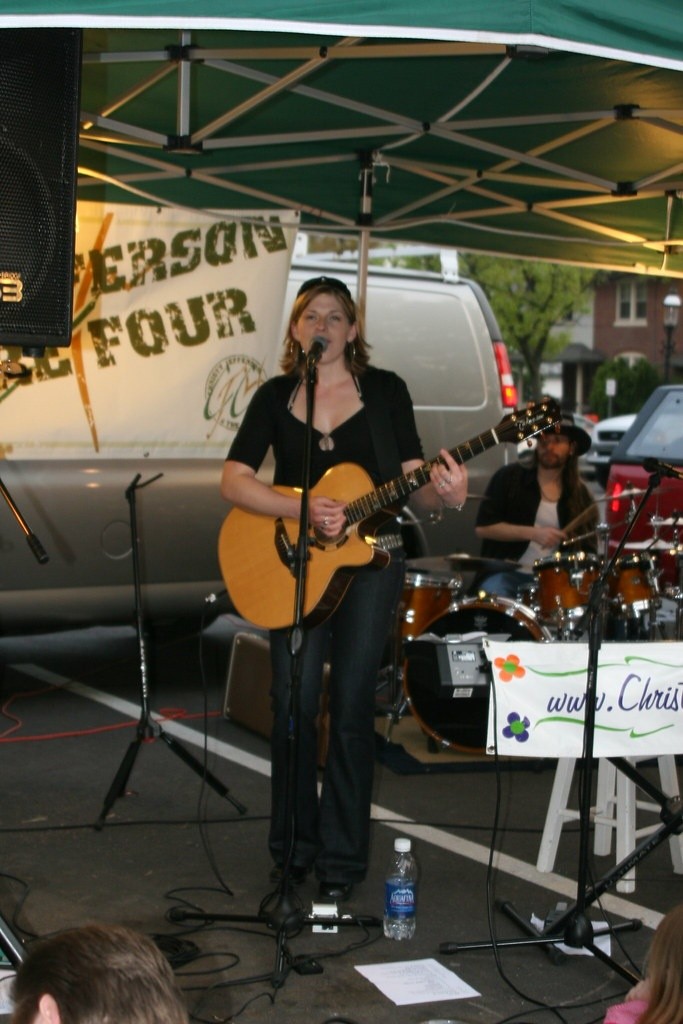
169;361;383;987
440;474;660;987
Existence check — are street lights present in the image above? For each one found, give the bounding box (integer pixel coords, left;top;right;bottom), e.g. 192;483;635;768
661;289;683;384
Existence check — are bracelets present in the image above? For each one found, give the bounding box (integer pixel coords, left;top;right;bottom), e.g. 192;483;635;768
443;502;464;511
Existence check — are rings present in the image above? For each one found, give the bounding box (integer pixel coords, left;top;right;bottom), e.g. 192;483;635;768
439;481;445;487
448;477;452;483
324;516;328;524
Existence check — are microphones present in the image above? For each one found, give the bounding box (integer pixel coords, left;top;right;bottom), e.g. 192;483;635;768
307;335;327;368
642;457;683;479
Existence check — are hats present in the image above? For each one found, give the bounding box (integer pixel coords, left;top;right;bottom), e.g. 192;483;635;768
529;412;592;456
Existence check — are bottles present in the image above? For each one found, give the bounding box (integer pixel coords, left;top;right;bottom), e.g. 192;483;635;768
383;838;419;940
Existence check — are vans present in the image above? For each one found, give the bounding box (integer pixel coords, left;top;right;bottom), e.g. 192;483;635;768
0;261;520;635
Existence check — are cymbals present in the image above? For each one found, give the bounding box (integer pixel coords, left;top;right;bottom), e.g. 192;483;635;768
590;488;672;502
646;516;683;525
623;538;675;551
404;553;524;576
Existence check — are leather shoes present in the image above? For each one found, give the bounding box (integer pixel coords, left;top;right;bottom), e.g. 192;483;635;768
271;845;315;885
318;864;354;903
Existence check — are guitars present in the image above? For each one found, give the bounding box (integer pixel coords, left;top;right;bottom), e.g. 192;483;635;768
218;396;564;635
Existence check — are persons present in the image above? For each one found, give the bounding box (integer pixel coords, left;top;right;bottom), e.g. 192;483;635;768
472;414;599;601
10;924;189;1024
602;904;683;1024
222;276;466;899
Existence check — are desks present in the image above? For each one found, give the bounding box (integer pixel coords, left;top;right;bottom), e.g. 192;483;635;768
483;639;683;892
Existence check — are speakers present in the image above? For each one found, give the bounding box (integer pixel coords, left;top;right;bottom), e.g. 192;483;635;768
0;28;83;346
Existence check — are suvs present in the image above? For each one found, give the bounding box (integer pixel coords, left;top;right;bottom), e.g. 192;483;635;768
571;384;683;586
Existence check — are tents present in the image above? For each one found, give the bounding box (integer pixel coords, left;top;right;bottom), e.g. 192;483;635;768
0;0;683;344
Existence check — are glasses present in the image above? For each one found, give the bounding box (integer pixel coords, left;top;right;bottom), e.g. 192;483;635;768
297;277;353;306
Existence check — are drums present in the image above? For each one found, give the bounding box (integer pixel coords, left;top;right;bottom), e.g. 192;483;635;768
510;583;538;611
402;595;549;752
599;551;663;623
399;571;464;641
532;551;600;630
